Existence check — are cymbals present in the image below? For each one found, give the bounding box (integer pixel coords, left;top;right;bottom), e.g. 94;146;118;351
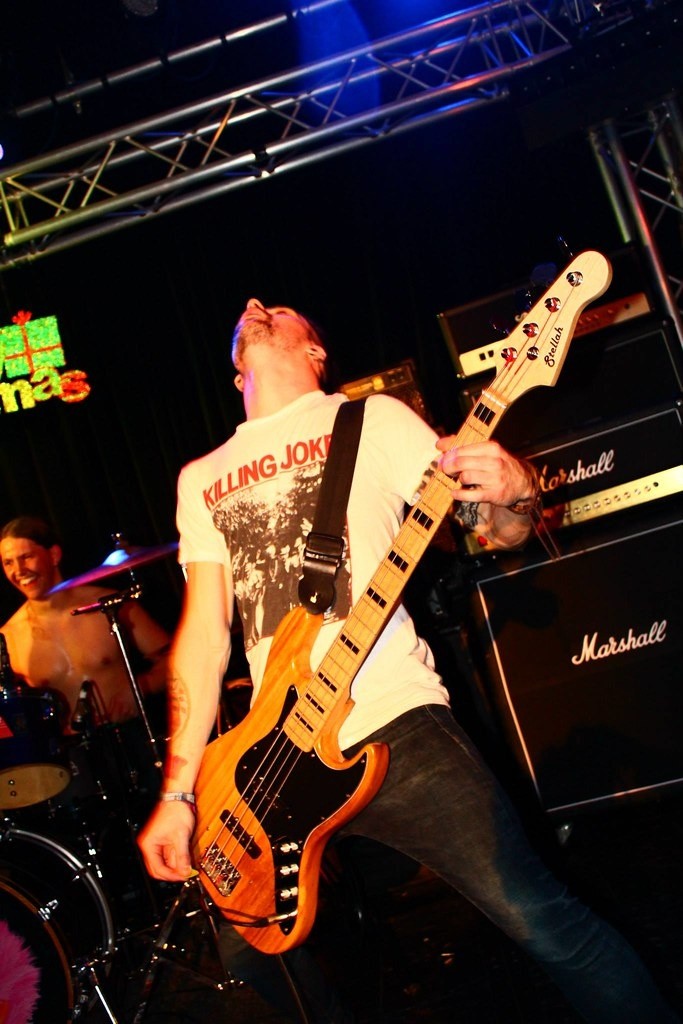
44;534;179;597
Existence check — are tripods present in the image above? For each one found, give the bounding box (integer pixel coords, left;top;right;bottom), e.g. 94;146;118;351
73;564;245;1024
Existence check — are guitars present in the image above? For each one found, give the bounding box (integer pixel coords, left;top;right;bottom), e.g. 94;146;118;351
186;249;614;956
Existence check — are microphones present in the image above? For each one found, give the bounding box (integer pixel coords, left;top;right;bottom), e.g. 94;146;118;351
72;678;92;731
84;834;102;876
0;633;21;700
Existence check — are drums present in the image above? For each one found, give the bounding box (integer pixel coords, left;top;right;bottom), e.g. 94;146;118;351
1;827;115;1024
1;688;85;812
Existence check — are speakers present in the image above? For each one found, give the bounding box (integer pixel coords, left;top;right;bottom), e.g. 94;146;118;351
466;494;683;827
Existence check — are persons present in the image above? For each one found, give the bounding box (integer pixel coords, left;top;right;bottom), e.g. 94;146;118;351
137;300;683;1022
1;516;172;992
